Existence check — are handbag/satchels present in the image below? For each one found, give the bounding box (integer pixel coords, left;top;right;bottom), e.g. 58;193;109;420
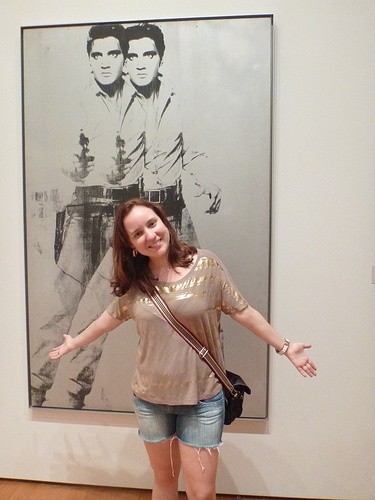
222;370;248;425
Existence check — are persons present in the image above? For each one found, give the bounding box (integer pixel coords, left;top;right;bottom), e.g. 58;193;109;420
49;197;319;498
22;23;226;409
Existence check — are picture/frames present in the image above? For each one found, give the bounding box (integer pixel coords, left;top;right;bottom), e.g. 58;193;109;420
20;13;272;420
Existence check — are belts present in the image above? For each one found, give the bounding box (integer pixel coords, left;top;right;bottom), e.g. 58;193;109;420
73;185;138;200
138;186;181;204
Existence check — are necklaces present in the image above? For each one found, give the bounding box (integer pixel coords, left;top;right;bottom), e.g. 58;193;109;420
163;262;172;270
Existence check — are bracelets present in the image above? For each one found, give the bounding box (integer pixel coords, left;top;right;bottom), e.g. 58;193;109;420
276;338;290;355
